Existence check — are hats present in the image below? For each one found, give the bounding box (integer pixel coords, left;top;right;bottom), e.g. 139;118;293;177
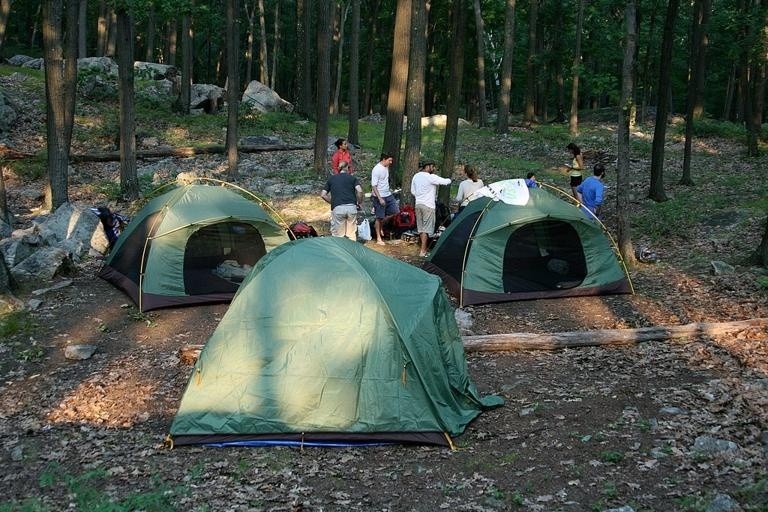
419;159;435;171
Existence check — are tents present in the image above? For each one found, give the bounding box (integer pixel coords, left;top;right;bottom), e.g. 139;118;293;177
422;175;636;309
165;227;508;451
98;174;294;315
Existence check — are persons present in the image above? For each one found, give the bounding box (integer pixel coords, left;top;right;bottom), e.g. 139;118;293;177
320;159;362;243
574;162;606;221
564;142;582;200
371;151;400;247
331;138;352;176
410;158;458;257
524;172;543;189
455;164;484;215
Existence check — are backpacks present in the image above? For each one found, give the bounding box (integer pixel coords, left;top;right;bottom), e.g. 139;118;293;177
287;221;318;241
